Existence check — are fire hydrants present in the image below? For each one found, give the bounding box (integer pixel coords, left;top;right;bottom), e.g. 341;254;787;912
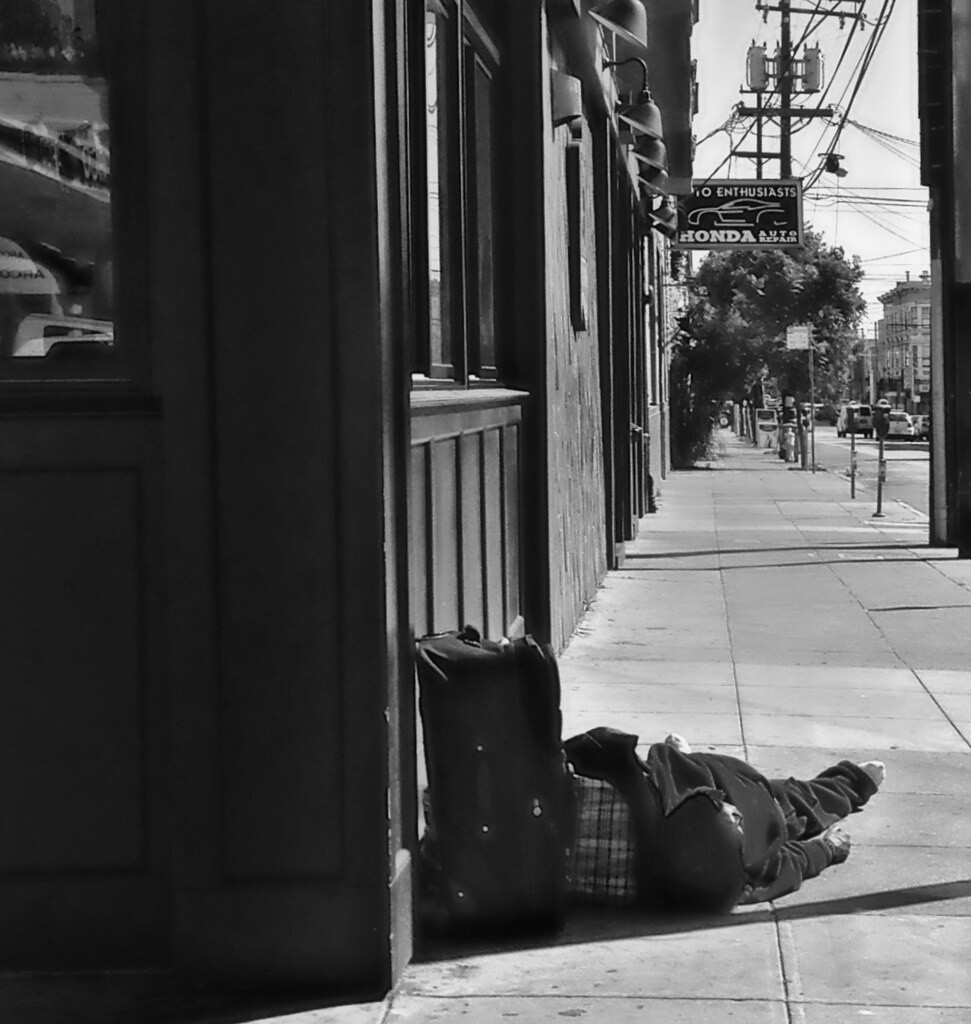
784;428;796;462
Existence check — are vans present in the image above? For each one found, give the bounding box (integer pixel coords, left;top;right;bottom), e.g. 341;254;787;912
835;404;874;439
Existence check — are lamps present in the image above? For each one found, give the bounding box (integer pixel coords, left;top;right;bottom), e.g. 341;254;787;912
587;0;679;240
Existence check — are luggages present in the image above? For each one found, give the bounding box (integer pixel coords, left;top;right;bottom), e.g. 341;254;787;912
411;620;570;951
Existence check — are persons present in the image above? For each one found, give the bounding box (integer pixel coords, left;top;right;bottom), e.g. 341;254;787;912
561;726;887;923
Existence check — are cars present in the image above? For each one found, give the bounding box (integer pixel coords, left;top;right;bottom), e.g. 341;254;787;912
909;414;930;441
874;410;915;442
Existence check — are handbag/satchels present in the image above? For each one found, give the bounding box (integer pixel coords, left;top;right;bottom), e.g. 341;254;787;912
567;762;646;910
